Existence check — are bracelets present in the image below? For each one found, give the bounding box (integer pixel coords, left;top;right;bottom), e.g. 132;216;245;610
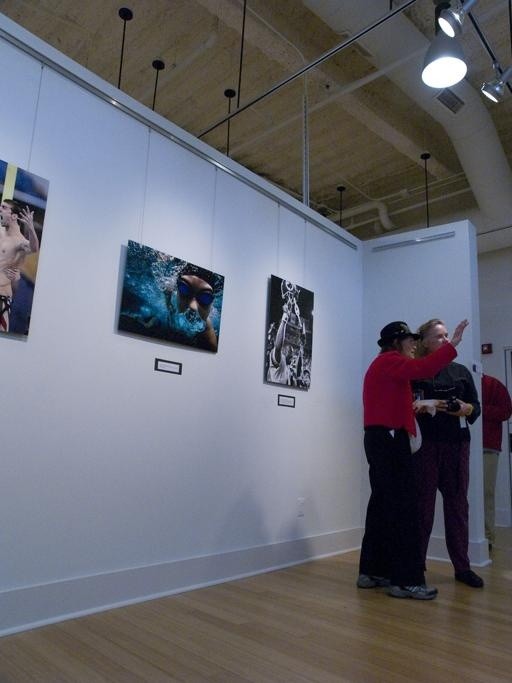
452;338;457;345
466;403;472;415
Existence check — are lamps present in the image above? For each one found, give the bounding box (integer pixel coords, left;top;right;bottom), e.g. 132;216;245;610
418;1;511;104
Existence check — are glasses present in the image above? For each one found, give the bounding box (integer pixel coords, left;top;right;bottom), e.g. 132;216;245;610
177;278;214;306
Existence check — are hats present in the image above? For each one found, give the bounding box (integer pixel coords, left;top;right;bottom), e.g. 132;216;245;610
179;263;214;289
378;321;420;346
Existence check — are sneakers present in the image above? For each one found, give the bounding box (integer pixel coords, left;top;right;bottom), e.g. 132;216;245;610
357;574;437;599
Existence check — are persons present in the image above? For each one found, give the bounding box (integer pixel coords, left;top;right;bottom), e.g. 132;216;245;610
482;372;512;551
141;263;218;350
267;278;311;387
1;198;40;332
4;267;21;297
407;318;483;587
356;318;469;600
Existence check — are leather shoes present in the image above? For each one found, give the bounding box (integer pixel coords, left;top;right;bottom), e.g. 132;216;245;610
455;570;483;587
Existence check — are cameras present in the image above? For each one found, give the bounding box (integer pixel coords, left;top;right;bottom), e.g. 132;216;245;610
447;396;460;412
